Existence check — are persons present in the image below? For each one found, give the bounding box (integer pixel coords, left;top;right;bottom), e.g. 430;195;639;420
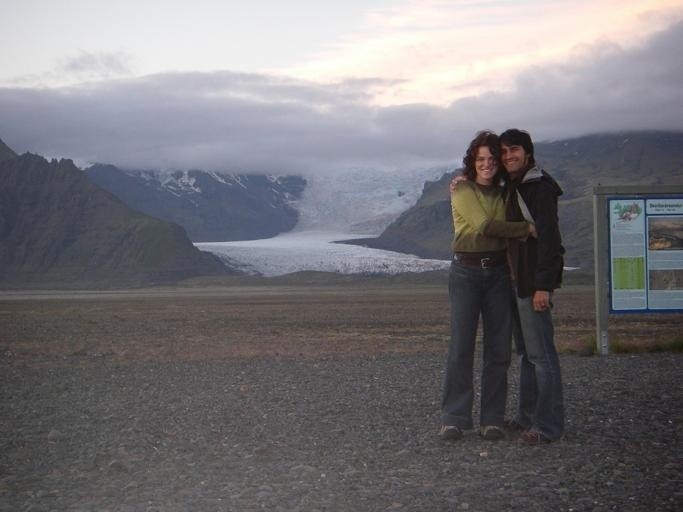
440;131;539;442
449;128;566;442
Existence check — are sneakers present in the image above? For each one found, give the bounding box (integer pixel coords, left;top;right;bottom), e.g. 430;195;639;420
517;433;552;446
503;418;528;432
479;425;505;439
438;425;463;439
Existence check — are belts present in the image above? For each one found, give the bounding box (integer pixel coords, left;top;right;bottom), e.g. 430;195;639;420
453;253;507;268
